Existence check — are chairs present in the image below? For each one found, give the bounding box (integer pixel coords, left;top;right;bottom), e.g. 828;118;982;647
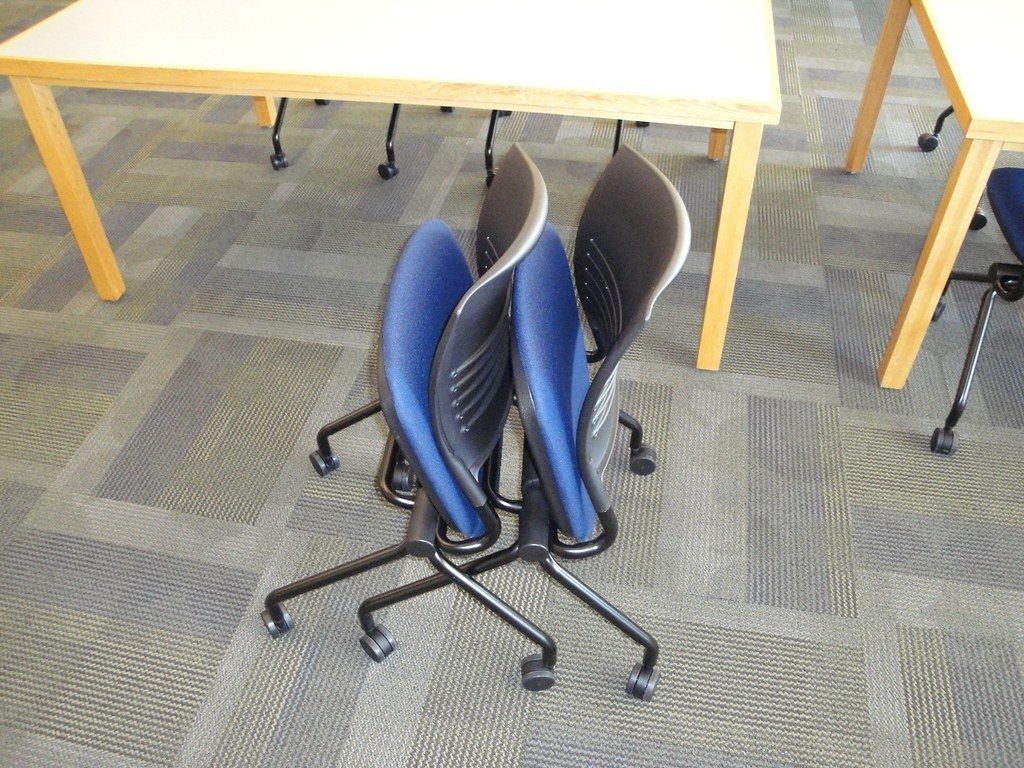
930;166;1024;454
259;141;691;700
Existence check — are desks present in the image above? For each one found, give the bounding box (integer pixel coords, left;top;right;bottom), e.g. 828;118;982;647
845;0;1024;390
0;0;781;372
